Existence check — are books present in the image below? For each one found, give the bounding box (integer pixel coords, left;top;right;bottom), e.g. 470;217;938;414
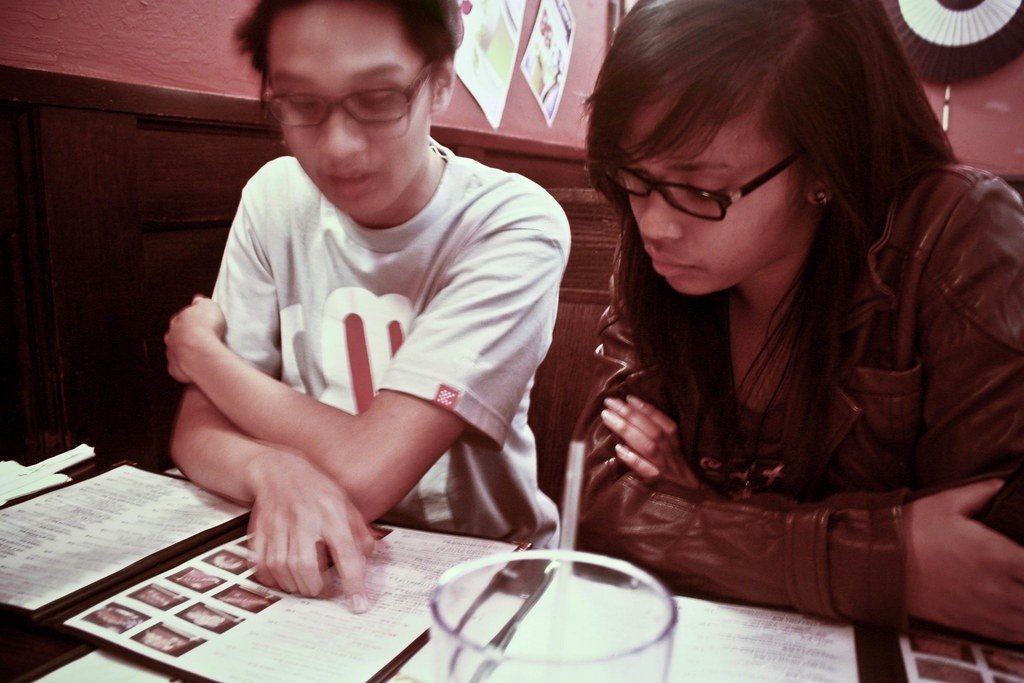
0;461;532;683
469;567;1024;683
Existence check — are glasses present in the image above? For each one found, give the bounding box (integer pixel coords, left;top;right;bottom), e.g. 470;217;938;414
603;150;806;221
260;64;433;127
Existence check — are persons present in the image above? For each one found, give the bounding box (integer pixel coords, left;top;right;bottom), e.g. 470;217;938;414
164;0;571;614
564;0;1024;644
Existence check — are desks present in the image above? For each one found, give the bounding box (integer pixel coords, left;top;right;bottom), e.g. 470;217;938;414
0;446;1024;683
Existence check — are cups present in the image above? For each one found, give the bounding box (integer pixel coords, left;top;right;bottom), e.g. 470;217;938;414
428;550;676;683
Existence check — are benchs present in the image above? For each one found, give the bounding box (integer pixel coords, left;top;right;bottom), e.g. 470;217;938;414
526;170;1024;515
